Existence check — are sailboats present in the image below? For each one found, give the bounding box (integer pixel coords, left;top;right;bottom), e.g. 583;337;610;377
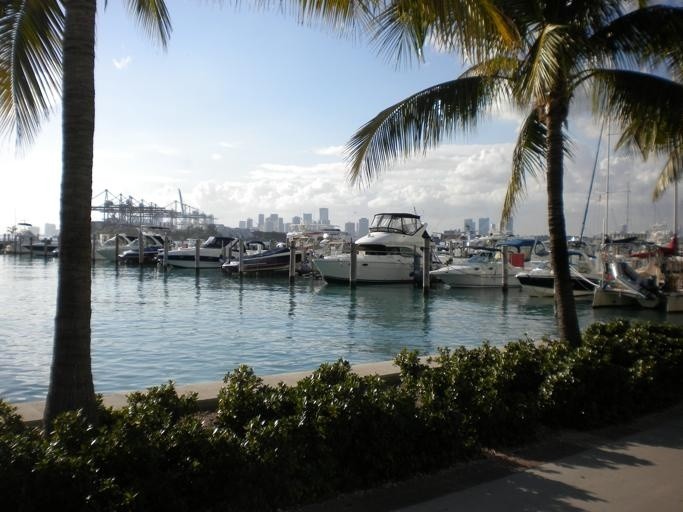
514;113;683;312
